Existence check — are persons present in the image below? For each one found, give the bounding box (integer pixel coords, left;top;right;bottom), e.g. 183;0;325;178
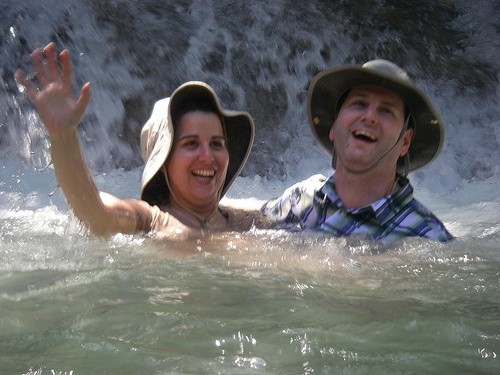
15;43;254;235
259;60;453;243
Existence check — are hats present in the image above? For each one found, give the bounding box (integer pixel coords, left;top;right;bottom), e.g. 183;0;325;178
139;80;257;209
307;59;444;175
